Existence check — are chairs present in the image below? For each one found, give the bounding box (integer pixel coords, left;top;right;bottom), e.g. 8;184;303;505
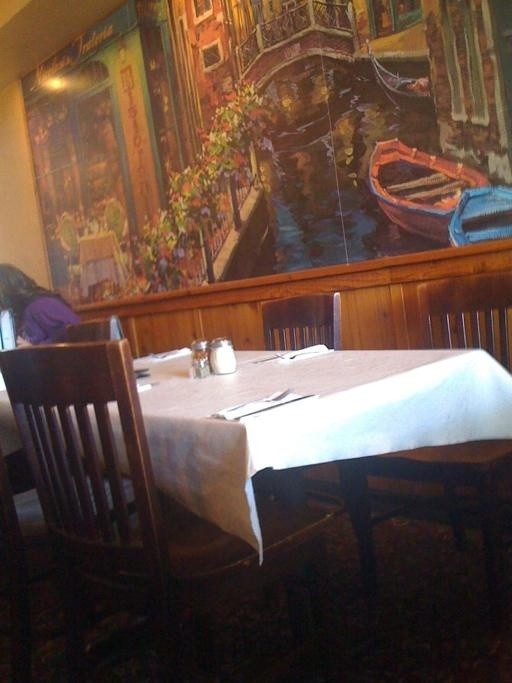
341;266;512;599
257;290;342;508
2;340;342;683
54;314;125;342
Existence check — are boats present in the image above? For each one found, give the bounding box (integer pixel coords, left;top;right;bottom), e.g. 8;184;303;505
369;54;434;111
368;137;512;246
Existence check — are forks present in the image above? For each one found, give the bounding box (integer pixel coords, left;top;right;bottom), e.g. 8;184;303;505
215;386;291;416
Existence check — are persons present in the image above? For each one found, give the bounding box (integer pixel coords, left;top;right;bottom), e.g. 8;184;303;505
0;263;80;349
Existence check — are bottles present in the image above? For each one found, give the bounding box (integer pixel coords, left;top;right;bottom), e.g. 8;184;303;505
190;337;237;378
